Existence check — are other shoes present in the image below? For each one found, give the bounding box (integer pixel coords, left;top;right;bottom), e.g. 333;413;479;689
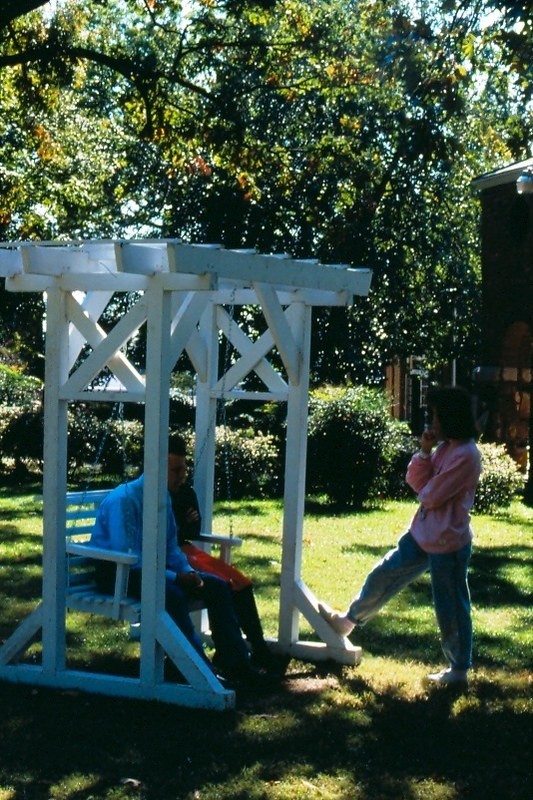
428;669;468;685
319;602;349;638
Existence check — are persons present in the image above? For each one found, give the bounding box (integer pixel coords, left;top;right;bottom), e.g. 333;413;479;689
91;437;283;692
319;387;481;684
